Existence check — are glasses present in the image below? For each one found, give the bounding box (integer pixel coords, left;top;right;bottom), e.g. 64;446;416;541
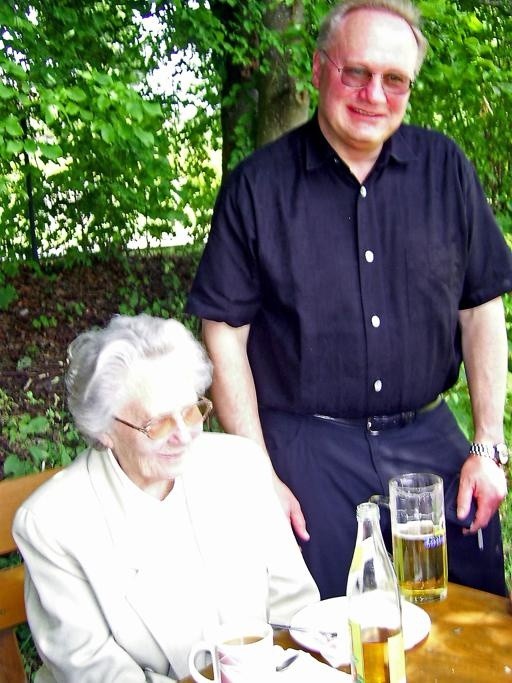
322;48;415;94
112;396;213;441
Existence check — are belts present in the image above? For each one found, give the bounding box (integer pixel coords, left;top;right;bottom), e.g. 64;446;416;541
312;393;443;432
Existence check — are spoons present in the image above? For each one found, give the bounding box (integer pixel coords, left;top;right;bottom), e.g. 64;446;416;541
276;653;301;673
265;618;349;641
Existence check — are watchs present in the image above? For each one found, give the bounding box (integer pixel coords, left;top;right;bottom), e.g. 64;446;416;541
466;437;510;468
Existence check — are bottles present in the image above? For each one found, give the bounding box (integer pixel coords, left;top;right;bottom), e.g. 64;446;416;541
346;501;406;683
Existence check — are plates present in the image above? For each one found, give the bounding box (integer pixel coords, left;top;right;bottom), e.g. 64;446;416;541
285;595;432;655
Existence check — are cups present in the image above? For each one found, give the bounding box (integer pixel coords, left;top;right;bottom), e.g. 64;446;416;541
367;471;448;603
188;623;276;683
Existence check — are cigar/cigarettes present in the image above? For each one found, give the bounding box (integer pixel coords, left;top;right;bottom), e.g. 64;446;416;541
476;526;485;551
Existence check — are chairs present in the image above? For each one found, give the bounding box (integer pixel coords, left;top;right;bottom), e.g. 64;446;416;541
0;465;82;683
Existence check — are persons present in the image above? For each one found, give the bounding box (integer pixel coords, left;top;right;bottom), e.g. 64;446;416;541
181;0;512;606
11;310;321;682
